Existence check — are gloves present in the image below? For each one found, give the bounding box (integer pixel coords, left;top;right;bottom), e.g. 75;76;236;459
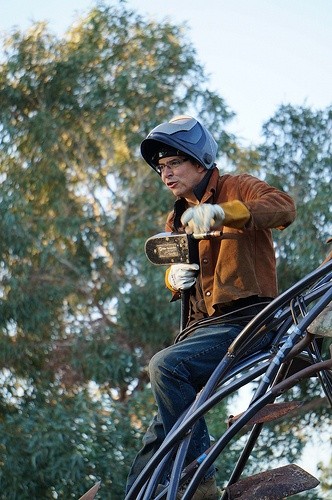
165;262;199;293
180;199;251;236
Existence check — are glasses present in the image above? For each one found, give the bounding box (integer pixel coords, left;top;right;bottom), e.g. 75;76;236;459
155;159;187;173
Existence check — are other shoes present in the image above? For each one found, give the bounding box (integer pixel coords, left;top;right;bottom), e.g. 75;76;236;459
175;475;219;500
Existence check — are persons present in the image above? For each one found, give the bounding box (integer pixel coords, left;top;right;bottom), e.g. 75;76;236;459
126;116;296;500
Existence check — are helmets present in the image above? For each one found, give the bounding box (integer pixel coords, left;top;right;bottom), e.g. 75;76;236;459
141;118;218;175
